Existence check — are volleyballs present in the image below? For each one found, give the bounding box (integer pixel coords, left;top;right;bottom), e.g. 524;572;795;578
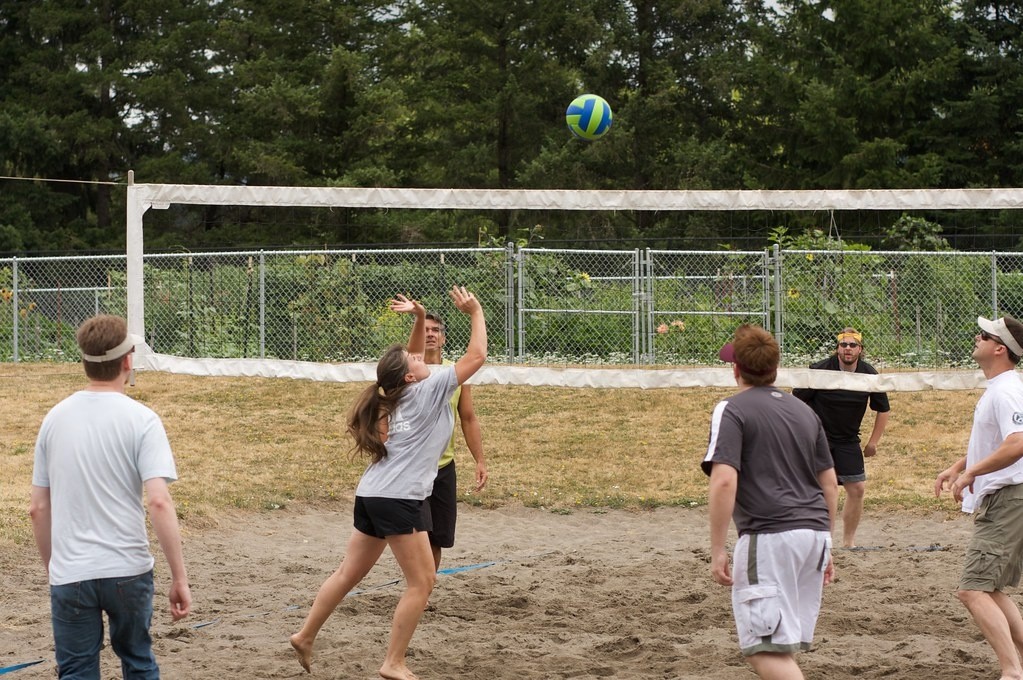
564;92;614;143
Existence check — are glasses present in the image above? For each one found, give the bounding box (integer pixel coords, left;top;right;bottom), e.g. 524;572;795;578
838;342;861;348
980;329;1005;345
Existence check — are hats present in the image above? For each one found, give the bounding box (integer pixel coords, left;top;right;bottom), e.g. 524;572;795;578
837;333;863;344
977;315;1023;357
78;333;145;363
720;343;777;376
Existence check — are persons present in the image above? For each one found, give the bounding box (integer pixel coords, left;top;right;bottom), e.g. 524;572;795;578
792;327;890;551
29;314;192;680
935;316;1023;680
700;326;839;680
290;285;487;680
378;315;487;611
